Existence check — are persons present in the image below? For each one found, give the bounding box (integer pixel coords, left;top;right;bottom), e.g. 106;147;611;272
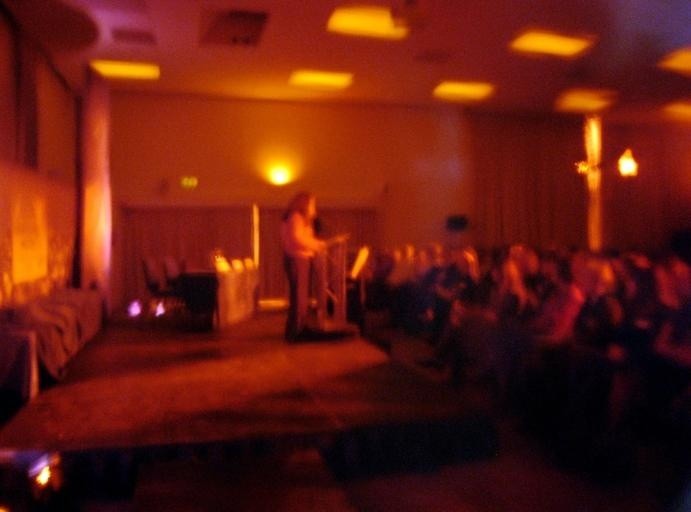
281;190;325;343
379;245;690;502
